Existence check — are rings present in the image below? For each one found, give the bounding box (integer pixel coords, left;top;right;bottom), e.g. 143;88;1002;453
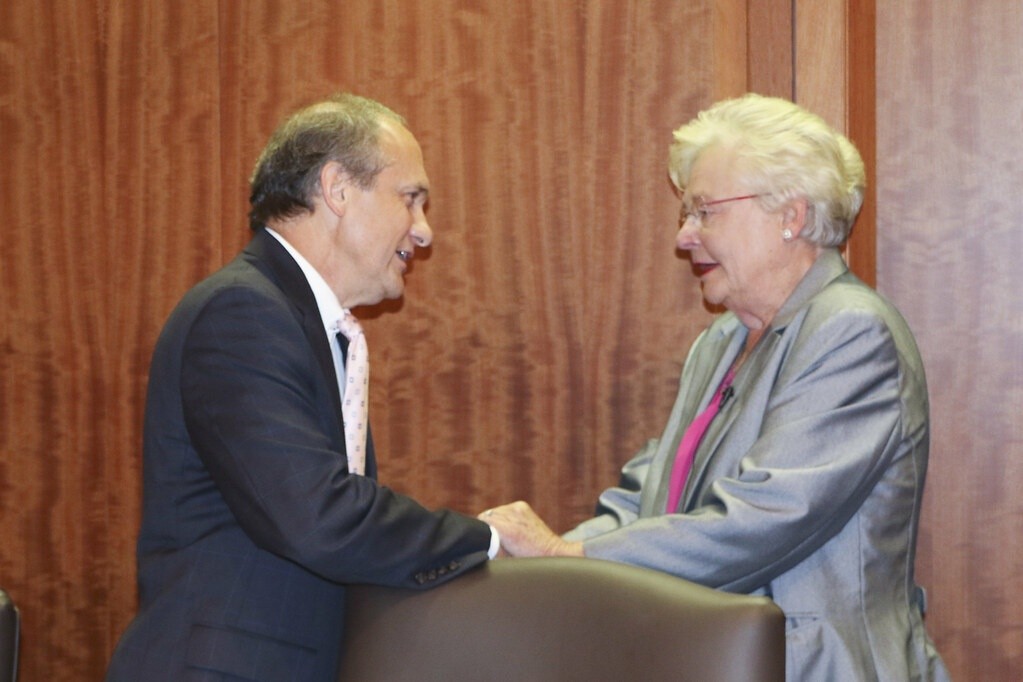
485;508;493;517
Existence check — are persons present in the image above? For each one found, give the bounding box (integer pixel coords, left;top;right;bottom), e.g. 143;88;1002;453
100;88;514;682
475;90;949;682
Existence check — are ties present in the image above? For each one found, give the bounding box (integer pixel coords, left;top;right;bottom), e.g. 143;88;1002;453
338;308;370;476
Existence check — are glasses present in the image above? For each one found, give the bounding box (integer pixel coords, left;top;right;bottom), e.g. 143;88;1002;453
679;192;775;227
